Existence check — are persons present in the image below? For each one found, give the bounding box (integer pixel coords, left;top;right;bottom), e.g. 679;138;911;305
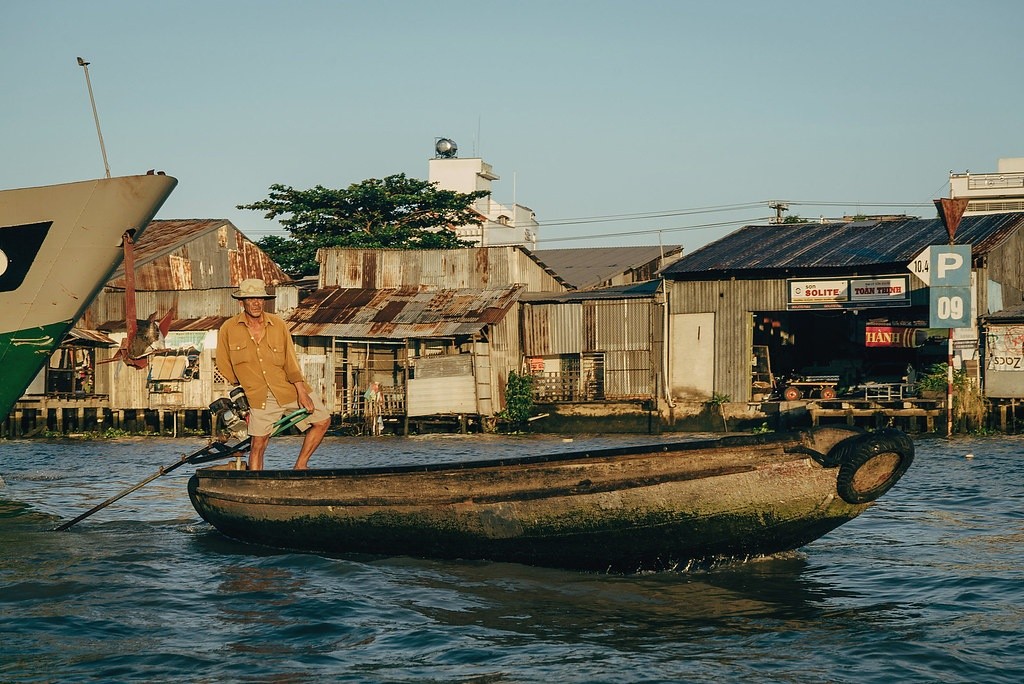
216;278;331;470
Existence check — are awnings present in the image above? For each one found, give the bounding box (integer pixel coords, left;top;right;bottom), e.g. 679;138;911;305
61;330;120;344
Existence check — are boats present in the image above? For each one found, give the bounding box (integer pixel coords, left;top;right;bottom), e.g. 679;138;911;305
188;426;914;574
0;168;178;431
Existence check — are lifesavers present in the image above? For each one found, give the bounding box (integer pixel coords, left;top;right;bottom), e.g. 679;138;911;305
834;430;915;503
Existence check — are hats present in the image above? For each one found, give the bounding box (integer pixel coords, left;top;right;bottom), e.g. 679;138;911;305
230;279;277;300
